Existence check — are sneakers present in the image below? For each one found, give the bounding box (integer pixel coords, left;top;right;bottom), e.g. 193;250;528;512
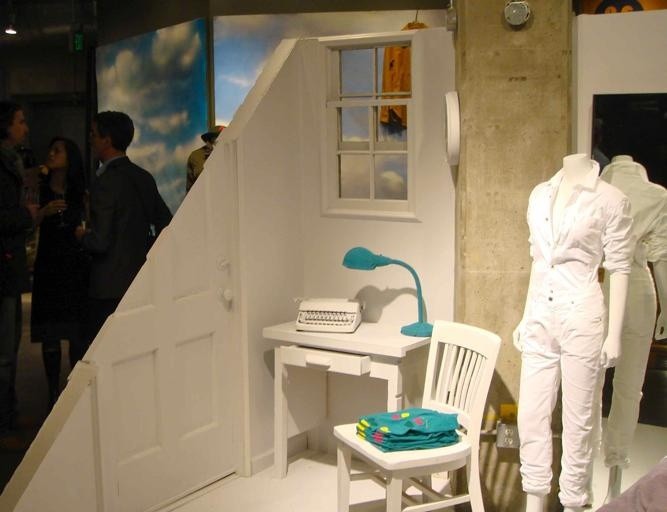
1;436;27;451
11;414;34;429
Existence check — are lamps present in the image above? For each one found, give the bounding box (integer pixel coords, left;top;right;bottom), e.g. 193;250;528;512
343;246;435;339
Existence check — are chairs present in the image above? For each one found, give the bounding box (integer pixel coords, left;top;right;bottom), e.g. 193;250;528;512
332;318;503;512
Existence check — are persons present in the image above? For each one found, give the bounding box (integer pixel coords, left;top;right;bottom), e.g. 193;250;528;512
2;100;41;415
510;153;633;512
184;125;229;195
61;110;173;352
30;137;90;402
601;153;667;512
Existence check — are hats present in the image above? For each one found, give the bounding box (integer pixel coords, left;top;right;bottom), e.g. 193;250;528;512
201;126;225;142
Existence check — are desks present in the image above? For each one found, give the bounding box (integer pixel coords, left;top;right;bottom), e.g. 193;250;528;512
258;317;437;482
